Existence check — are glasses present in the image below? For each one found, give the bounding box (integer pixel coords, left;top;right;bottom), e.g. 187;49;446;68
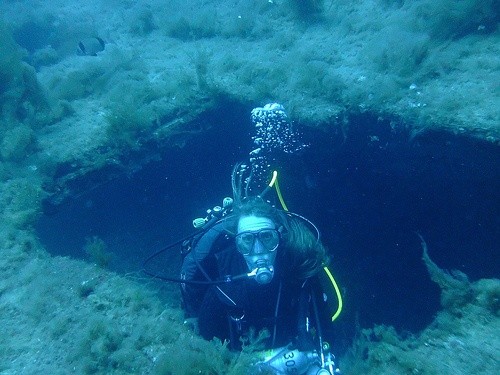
232;228;281;256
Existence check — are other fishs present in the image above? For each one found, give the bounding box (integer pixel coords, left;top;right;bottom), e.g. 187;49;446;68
74;36;104;58
408;82;428;109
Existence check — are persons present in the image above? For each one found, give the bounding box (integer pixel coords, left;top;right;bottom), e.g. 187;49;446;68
179;203;339;371
